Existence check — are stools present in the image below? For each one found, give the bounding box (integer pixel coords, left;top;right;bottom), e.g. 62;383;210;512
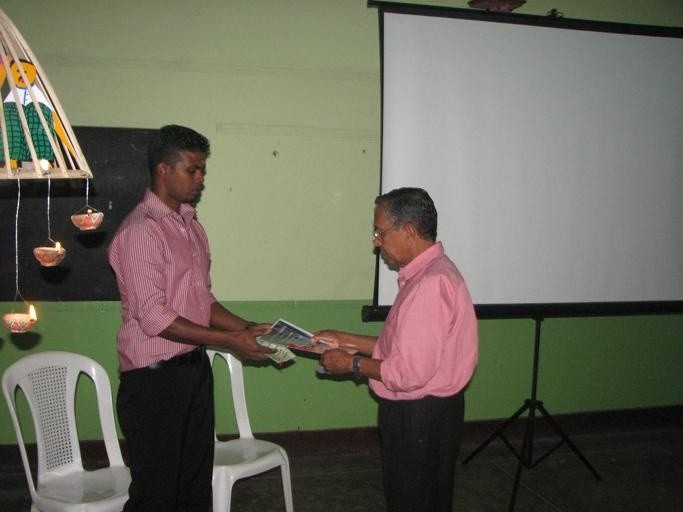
144;346;206;370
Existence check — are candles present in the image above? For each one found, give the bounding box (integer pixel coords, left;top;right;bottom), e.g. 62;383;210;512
2;206;112;333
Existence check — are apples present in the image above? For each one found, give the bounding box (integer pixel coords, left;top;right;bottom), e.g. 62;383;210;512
2;206;112;333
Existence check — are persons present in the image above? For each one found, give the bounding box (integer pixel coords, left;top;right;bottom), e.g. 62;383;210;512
106;123;277;509
315;185;480;510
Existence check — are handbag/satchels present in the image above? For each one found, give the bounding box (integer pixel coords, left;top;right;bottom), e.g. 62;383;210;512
352;351;366;379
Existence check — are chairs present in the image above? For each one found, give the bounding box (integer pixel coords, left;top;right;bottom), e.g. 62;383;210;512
2;350;132;510
205;347;293;508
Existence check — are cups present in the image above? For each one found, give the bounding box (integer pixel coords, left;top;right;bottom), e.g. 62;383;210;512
374;229;388;239
